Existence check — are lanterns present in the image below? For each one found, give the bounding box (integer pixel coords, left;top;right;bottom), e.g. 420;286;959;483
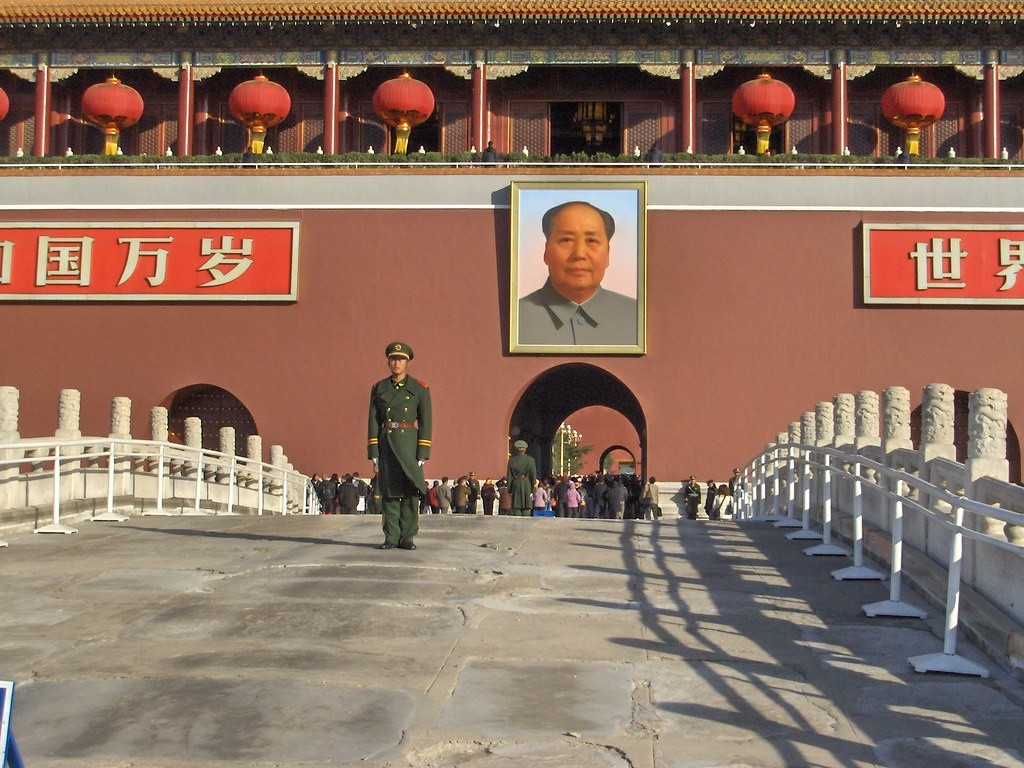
880;76;945;155
731;74;795;154
81;78;144;156
373;75;434;154
0;87;9;121
229;76;291;154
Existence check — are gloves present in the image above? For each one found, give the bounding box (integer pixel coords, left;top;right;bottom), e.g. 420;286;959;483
417;457;426;467
372;456;378;465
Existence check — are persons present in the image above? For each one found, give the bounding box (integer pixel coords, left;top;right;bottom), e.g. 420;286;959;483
519;201;637;346
897;148;914;169
646;143;664;168
684;476;701;520
306;463;381;514
704;468;744;521
507;440;537;516
242;147;258;169
482;141;499;168
420;472;513;515
530;473;658;520
368;343;432;551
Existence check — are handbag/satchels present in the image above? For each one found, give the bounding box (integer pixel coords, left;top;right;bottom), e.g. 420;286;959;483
714;509;720;517
644;497;654;506
657;507;662;517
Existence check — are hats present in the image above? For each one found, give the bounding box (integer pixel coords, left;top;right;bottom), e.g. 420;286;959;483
470;472;476;476
690;475;696;480
514;440;528;451
733;468;740;473
706;480;714;485
385;342;414;362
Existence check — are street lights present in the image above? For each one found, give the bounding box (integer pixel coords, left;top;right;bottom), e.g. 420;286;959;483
551;421;583;478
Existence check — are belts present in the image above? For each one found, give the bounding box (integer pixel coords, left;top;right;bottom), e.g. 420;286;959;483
380;422;415;428
515;476;529;480
689;493;699;496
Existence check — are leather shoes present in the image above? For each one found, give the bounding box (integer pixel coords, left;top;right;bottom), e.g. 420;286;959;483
381;542;397;548
399;542;417;550
